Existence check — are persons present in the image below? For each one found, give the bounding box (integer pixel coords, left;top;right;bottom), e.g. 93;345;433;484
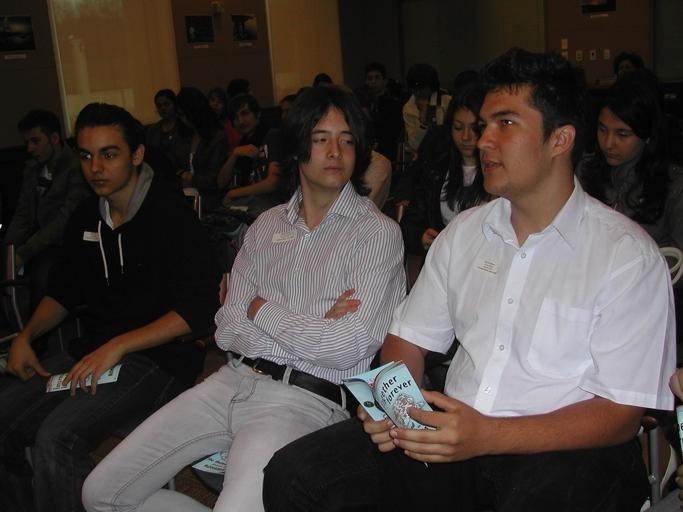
2;102;219;512
573;53;682;283
82;79;407;512
145;77;307;267
313;63;490;288
2;111;86;354
263;48;678;512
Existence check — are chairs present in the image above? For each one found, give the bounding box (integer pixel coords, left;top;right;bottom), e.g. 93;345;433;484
0;243;27;365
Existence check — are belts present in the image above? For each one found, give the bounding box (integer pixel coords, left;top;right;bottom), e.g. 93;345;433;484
232;353;358;413
